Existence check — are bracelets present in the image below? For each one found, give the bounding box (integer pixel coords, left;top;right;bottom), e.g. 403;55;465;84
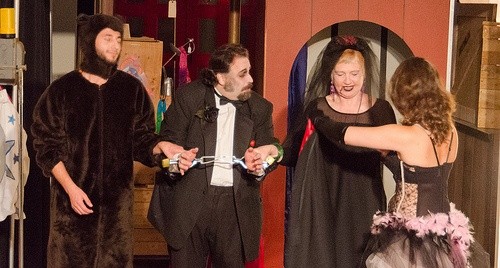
176;153;180;172
272;142;284;160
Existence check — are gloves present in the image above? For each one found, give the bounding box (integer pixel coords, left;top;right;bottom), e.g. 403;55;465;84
309;109;348;145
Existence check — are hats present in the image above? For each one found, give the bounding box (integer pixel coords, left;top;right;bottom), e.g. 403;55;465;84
77;14;124;80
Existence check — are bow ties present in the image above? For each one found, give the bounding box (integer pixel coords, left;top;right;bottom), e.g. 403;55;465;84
213;90;238;109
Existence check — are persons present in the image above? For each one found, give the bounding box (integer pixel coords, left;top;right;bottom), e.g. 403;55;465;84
31;13;185;268
158;44;280;268
307;57;491;268
252;36;398;268
